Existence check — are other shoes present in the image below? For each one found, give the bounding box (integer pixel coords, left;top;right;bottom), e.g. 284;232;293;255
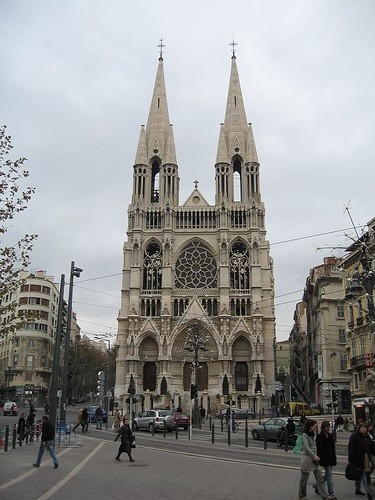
33;463;39;468
356;492;366;495
312;484;320;494
325;495;334;500
332;495;337;499
54;465;59;468
299;497;308;500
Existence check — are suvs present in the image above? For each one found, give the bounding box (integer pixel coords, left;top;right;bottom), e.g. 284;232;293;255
132;409;177;434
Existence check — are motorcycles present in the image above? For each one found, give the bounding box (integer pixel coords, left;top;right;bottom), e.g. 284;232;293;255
276;425;299;449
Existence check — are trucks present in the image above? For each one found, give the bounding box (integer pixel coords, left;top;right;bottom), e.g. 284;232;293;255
279;402;320;417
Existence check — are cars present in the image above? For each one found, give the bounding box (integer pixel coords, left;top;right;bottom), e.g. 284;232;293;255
215;408;257;421
3;401;20;416
251;417;306;446
86;406;109;424
174;412;190;430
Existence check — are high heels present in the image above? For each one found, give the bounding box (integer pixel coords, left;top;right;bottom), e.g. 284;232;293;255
116;457;121;461
131;460;135;462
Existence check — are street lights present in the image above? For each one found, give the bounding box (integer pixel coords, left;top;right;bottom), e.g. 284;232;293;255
56;259;83;436
183;334;209;428
93;336;110;411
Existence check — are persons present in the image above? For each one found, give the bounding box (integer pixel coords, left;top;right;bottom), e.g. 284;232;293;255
285;418;295;452
298;419;337;500
16;404;38;442
10;403;17;416
114;419;135;462
332;414;355;432
292;404;298;416
226;407;236;429
272;405;280;418
347;417;375;500
200;405;206;424
33;415;59;468
95;406;103;430
177;405;182;412
114;407;122;427
72;408;90;433
300;412;308;424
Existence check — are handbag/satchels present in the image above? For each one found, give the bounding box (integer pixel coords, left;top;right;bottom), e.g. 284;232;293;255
345;464;362;481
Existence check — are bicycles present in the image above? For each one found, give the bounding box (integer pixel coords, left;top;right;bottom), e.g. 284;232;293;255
222;414;240;433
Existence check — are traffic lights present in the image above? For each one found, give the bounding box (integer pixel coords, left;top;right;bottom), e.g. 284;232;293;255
326;402;332;408
332;389;338;402
333;403;338;408
190;383;198;399
